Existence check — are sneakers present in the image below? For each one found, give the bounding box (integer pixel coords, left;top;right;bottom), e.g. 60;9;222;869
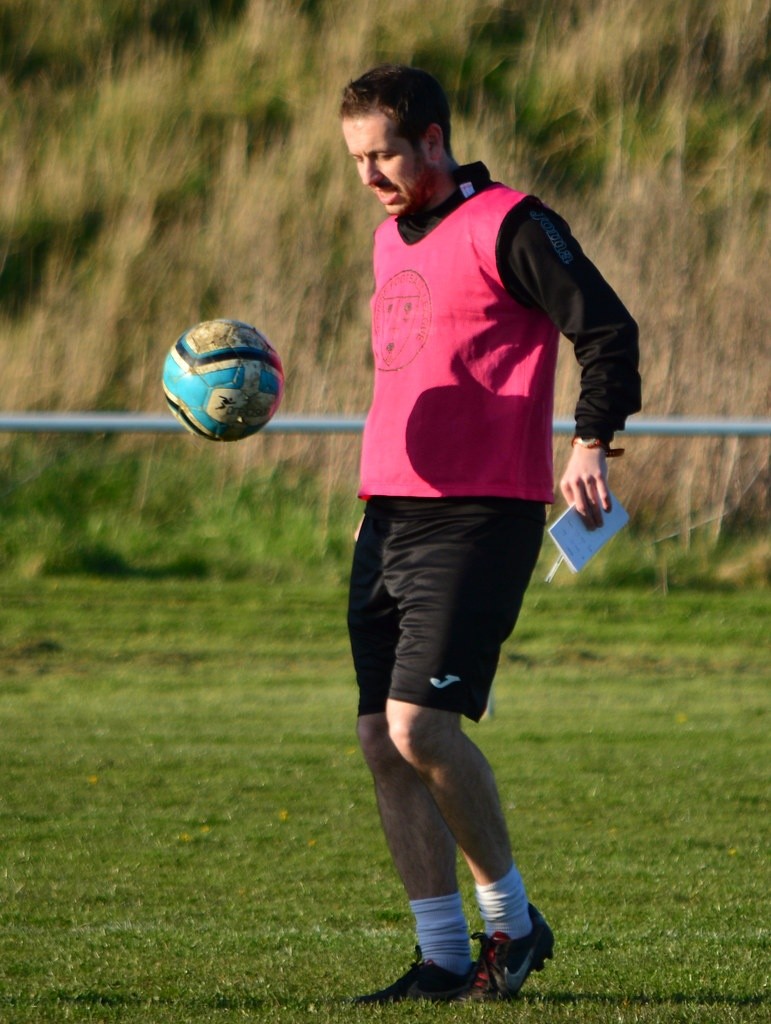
457;902;554;1003
355;946;477;1004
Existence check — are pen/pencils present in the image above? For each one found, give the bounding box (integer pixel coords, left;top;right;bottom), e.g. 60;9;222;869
543;553;565;584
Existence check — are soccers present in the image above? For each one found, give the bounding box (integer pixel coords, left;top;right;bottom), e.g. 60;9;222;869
161;317;287;444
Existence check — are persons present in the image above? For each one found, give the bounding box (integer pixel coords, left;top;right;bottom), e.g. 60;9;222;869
339;62;641;1007
